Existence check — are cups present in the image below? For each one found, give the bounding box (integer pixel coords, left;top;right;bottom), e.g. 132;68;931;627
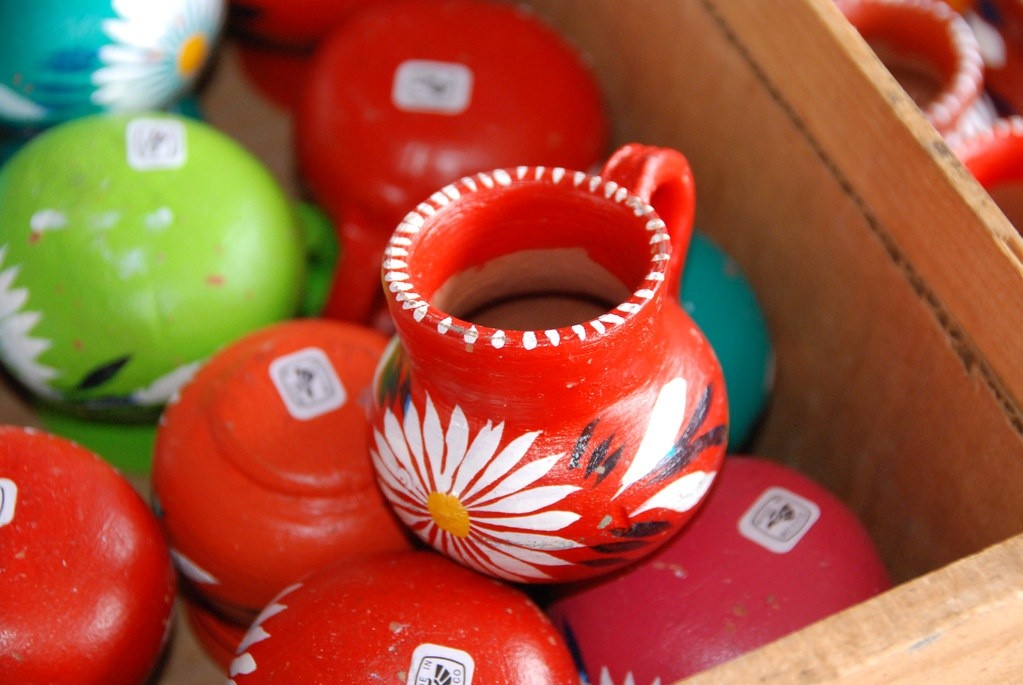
367;140;730;584
835;1;1002;165
